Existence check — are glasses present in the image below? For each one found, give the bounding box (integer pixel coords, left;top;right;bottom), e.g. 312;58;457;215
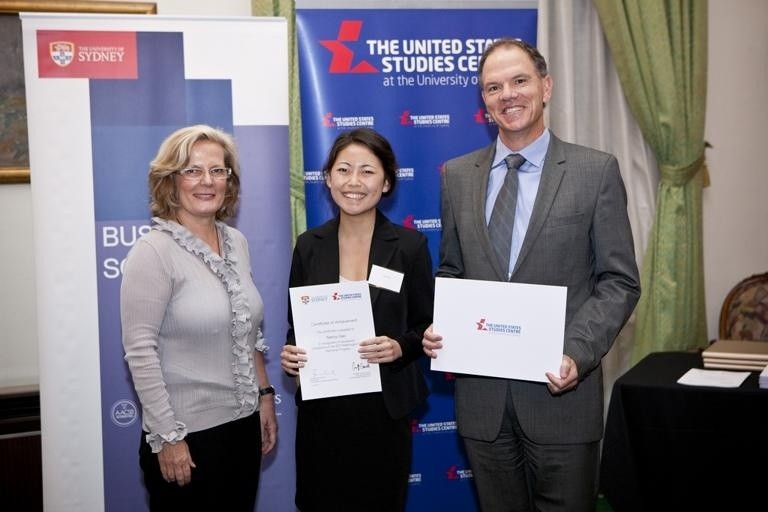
172;167;232;180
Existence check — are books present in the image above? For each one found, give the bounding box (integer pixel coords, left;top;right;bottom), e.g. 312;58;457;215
704;362;767;372
700;339;768;361
702;357;768;367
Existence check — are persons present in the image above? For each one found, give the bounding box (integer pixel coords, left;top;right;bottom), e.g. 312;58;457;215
117;122;280;511
278;125;434;511
422;36;644;511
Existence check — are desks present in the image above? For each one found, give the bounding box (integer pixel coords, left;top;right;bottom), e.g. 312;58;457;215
600;353;768;512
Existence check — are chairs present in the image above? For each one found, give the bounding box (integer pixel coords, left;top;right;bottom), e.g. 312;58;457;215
720;273;768;341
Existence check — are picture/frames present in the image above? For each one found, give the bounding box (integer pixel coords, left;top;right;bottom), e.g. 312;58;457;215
0;0;159;185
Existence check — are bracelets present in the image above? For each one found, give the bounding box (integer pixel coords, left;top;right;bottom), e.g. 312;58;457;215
258;384;277;397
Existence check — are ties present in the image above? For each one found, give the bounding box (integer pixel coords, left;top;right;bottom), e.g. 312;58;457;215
486;153;527;281
